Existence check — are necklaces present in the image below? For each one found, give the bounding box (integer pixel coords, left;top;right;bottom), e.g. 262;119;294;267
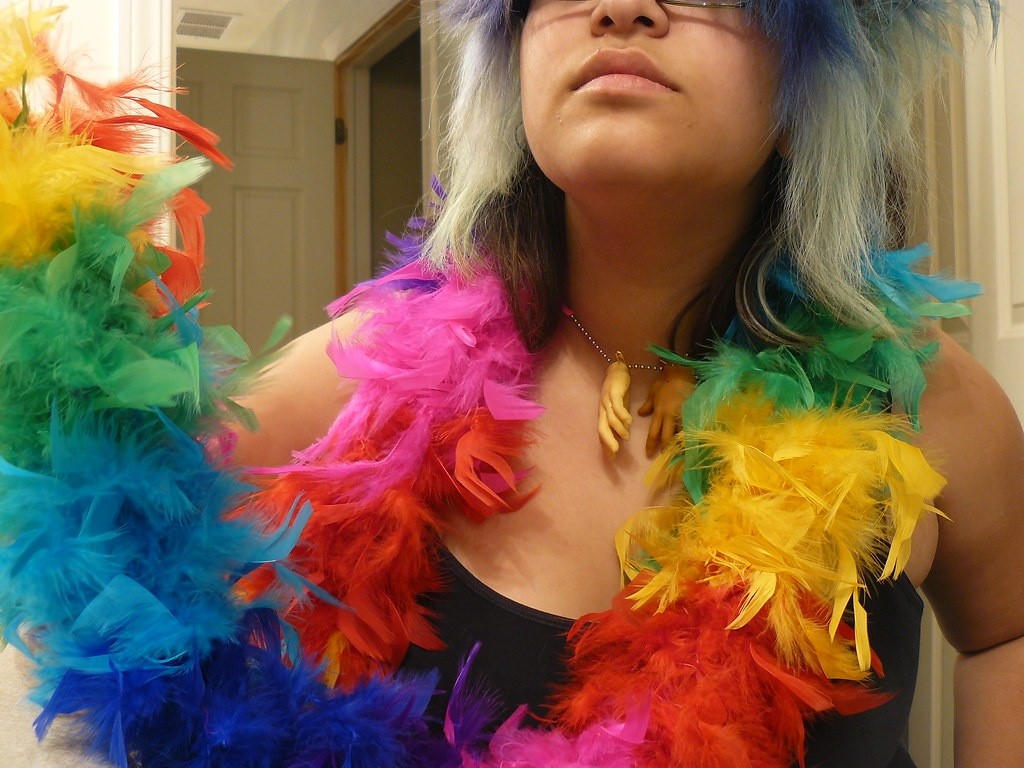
561;302;702;457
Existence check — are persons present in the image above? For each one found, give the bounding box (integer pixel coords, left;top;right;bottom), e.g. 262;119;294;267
0;0;1024;768
